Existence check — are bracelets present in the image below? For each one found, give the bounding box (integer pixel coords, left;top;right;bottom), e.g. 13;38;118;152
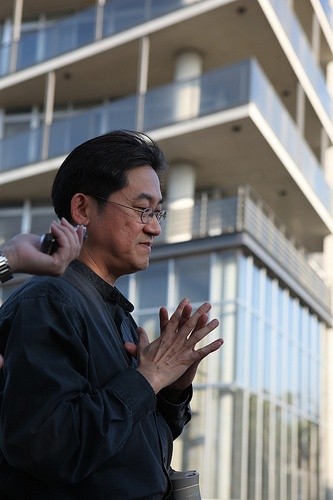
0;252;12;284
160;381;193;406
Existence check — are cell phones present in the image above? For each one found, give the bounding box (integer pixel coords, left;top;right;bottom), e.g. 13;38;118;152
41;224;87;251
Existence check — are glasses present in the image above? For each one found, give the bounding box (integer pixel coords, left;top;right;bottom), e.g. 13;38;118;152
76;191;167;224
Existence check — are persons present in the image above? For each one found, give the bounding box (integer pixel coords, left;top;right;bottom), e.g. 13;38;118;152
0;128;223;500
1;217;83;282
